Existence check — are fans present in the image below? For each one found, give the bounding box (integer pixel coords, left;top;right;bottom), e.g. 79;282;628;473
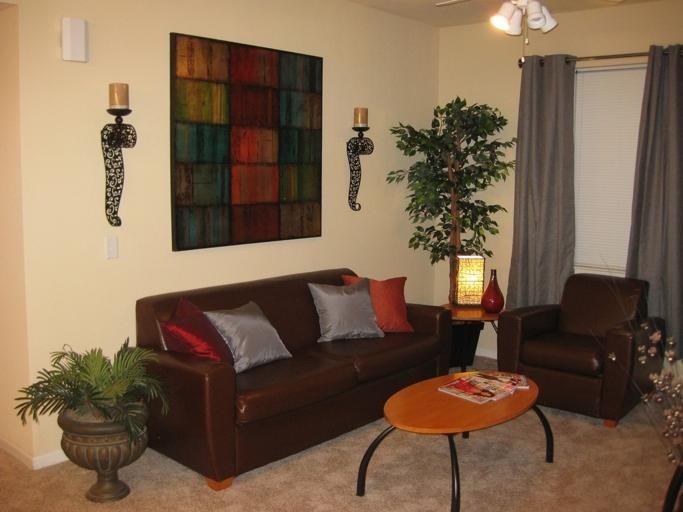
434;0;625;10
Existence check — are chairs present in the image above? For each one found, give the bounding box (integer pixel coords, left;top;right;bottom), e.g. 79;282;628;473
495;272;668;429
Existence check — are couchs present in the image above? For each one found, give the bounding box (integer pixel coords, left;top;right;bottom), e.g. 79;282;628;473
135;268;455;491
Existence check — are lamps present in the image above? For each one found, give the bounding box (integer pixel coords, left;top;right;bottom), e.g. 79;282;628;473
452;255;486;307
490;0;559;64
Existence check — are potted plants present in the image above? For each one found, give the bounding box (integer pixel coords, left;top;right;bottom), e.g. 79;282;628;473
13;337;170;503
385;96;520;364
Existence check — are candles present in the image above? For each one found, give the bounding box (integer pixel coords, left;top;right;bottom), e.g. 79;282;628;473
108;81;130;109
353;106;368;127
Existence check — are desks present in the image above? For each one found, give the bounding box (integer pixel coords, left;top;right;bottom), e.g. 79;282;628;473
440;305;508;371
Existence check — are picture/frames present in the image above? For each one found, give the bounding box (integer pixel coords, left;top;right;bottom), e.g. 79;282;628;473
169;31;324;252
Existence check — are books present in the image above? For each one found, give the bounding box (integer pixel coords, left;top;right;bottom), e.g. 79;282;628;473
438;368;531;407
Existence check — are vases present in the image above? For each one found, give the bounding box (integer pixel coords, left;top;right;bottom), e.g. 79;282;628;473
481;268;505;314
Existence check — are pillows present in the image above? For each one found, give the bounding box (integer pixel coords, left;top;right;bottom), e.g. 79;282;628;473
201;300;293;374
340;274;415;333
307;276;386;343
163;294;235;373
